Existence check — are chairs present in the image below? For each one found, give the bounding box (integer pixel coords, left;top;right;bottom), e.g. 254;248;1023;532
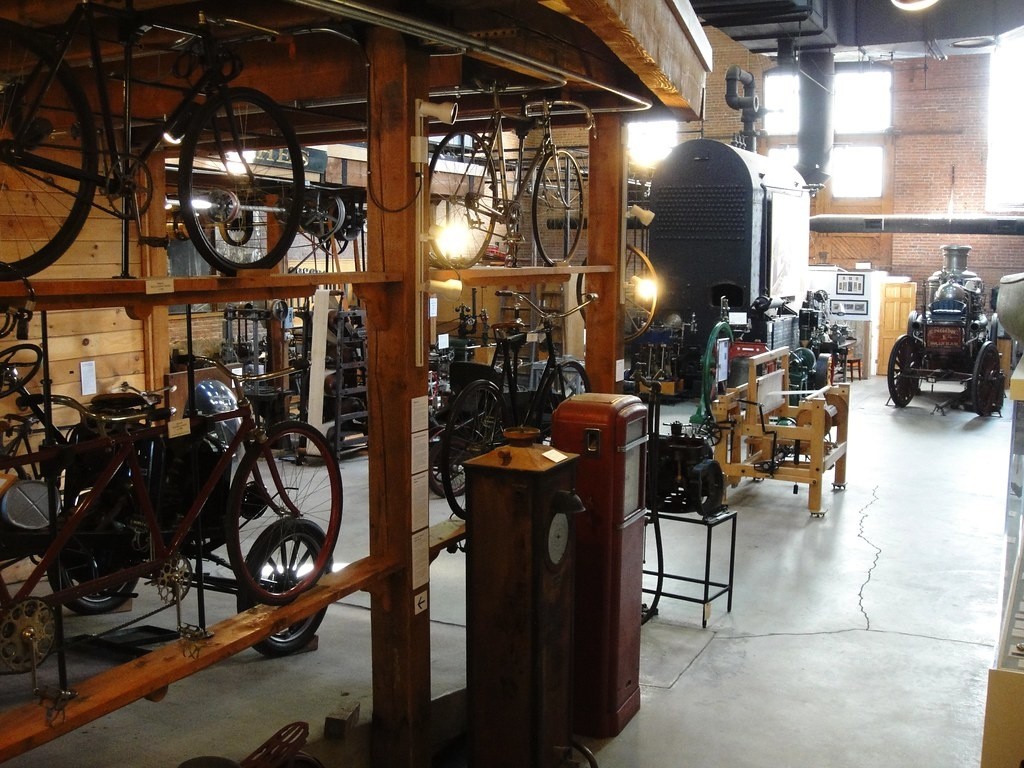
840;353;862;382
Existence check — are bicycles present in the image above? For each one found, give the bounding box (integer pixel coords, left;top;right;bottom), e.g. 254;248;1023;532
442;291;600;521
427;78;594;270
0;355;344;696
0;5;306;282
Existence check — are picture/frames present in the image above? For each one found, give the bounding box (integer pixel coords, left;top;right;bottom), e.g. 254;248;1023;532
830;300;868;315
836;274;865;295
